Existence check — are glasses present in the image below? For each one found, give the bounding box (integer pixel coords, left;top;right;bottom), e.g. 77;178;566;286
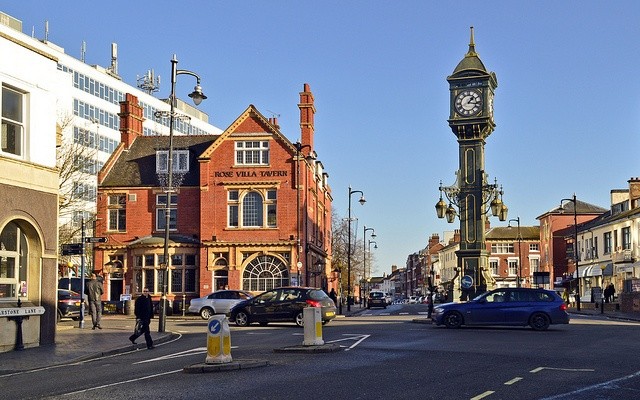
143;291;149;292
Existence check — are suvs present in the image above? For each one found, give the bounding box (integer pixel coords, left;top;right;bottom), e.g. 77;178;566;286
431;288;570;330
368;292;386;309
228;286;337;328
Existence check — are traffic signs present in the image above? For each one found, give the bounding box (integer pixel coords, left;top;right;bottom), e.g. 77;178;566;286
0;306;45;316
61;244;82;249
85;237;108;243
61;249;83;255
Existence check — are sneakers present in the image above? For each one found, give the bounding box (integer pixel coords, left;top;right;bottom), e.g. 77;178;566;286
92;325;96;329
96;324;102;329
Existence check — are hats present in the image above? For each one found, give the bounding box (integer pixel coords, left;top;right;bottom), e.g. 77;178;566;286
90;274;96;280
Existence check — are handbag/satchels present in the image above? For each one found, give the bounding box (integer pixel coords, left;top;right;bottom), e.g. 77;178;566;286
135;328;141;336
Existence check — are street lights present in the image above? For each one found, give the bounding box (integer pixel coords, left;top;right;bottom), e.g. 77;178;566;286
154;70;207;332
364;228;376;307
557;199;580;311
342;190;366;311
368;241;378;290
507;219;521;281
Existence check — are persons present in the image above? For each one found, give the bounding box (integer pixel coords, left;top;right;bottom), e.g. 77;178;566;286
609;283;615;303
84;273;104;330
604;285;611;303
128;287;155;349
562;289;570;307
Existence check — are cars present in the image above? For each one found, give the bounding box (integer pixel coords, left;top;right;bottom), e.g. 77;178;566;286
57;289;85;323
188;291;253;320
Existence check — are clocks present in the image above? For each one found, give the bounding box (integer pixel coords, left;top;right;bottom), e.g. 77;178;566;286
454;87;483;116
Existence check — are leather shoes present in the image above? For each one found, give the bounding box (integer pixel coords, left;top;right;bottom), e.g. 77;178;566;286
148;346;156;349
129;338;137;344
